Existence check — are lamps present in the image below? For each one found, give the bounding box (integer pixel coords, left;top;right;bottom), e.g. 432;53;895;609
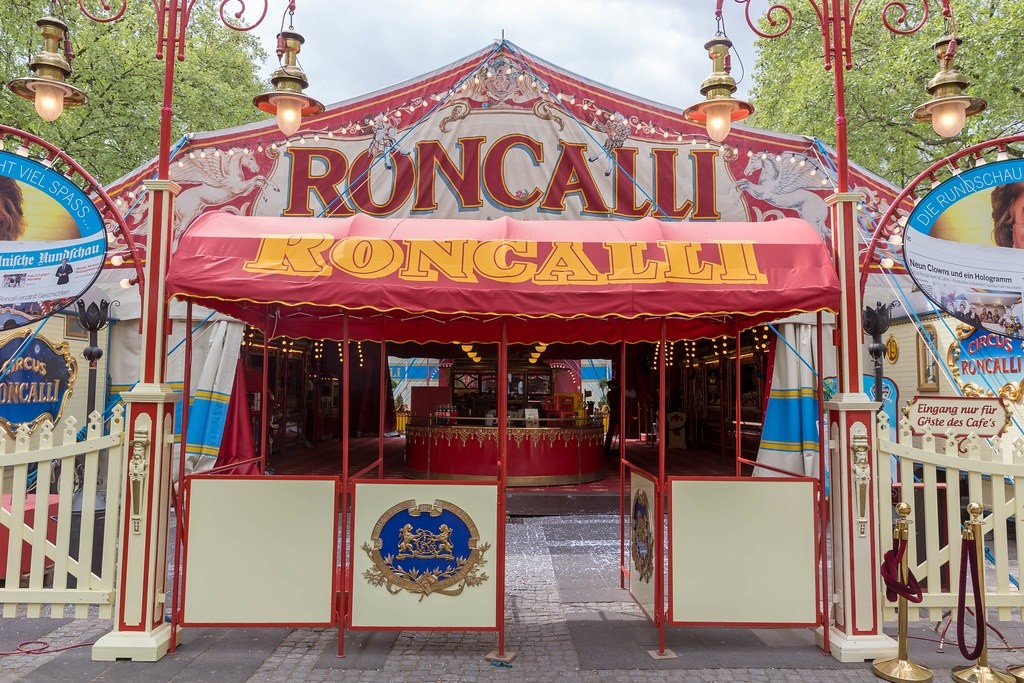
909;18;987;138
6;0;91;120
253;0;325;136
682;15;755;142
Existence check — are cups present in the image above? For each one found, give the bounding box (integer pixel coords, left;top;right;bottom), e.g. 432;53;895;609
507;410;523;418
485;413;498;427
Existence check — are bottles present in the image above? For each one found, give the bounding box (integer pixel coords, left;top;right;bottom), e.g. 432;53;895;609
435;404;457;425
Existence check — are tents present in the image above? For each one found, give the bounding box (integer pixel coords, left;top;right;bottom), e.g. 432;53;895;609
87;39;1024;514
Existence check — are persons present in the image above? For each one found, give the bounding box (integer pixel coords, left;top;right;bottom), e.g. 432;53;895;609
603;379;627;455
